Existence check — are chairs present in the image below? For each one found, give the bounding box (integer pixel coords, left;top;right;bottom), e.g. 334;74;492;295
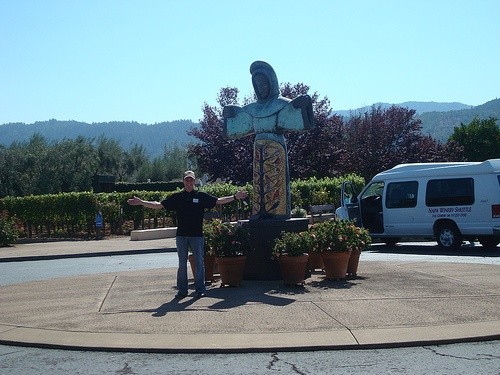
390;187;401;201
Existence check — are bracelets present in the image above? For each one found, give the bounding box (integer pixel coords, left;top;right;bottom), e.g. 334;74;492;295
234;194;237;200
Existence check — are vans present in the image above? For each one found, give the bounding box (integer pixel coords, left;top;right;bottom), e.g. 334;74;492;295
334;158;500;252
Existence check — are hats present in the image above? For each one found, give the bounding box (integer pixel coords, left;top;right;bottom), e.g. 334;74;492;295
183;171;195;180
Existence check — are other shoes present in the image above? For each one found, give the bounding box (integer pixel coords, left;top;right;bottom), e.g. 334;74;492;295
175;291;188;299
194;292;206;298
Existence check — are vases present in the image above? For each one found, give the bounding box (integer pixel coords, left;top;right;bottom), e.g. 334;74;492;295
308;251;323;269
347;251;362;275
188;255;246;287
321;251;351;280
278;254;309;283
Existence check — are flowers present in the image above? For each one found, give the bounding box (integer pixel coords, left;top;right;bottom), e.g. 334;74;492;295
187;220;244;253
272;218;372;253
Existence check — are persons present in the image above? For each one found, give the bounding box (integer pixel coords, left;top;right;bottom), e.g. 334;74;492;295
223;61;316;219
128;170;248;299
